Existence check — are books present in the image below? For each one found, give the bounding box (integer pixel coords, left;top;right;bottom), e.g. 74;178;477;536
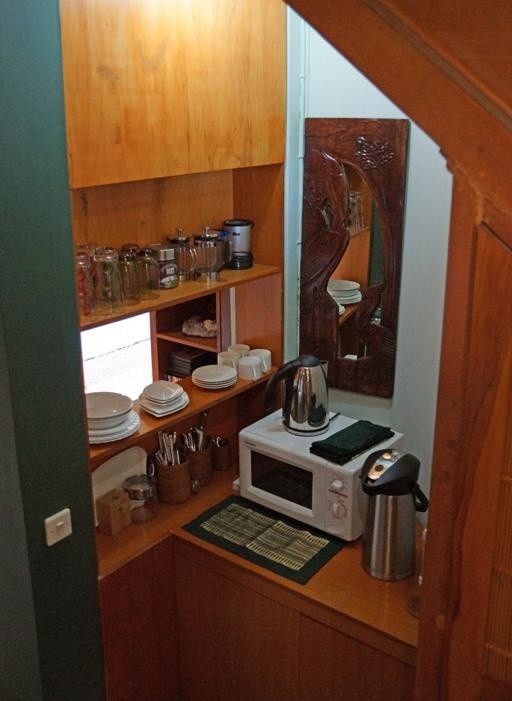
168;347;208;374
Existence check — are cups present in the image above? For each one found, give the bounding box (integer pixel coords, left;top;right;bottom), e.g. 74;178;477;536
155;443;231;504
216;343;273;382
168;227;226;282
122;475;156;526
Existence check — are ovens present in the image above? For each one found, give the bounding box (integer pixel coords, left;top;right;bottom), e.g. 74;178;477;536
238;401;405;545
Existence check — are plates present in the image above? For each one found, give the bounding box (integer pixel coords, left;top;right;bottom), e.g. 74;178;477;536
138;365;239;417
87;410;140;445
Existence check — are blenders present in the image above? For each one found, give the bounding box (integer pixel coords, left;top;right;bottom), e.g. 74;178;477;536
223;220;255;271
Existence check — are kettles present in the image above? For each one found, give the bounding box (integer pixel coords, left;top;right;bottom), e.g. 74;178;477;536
262;354;330;439
359;448;430;583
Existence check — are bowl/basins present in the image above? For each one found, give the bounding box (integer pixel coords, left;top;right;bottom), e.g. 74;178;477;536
87;392;134;430
326;278;361;292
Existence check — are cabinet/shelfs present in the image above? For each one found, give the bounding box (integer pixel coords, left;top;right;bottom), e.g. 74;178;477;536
99;535;178;701
59;0;295;571
174;537;423;701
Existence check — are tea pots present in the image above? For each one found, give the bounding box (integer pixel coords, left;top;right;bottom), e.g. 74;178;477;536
328;292;362;307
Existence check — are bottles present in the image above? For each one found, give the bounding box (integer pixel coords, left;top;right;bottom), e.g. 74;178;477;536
371;304;383;324
76;226;179;321
